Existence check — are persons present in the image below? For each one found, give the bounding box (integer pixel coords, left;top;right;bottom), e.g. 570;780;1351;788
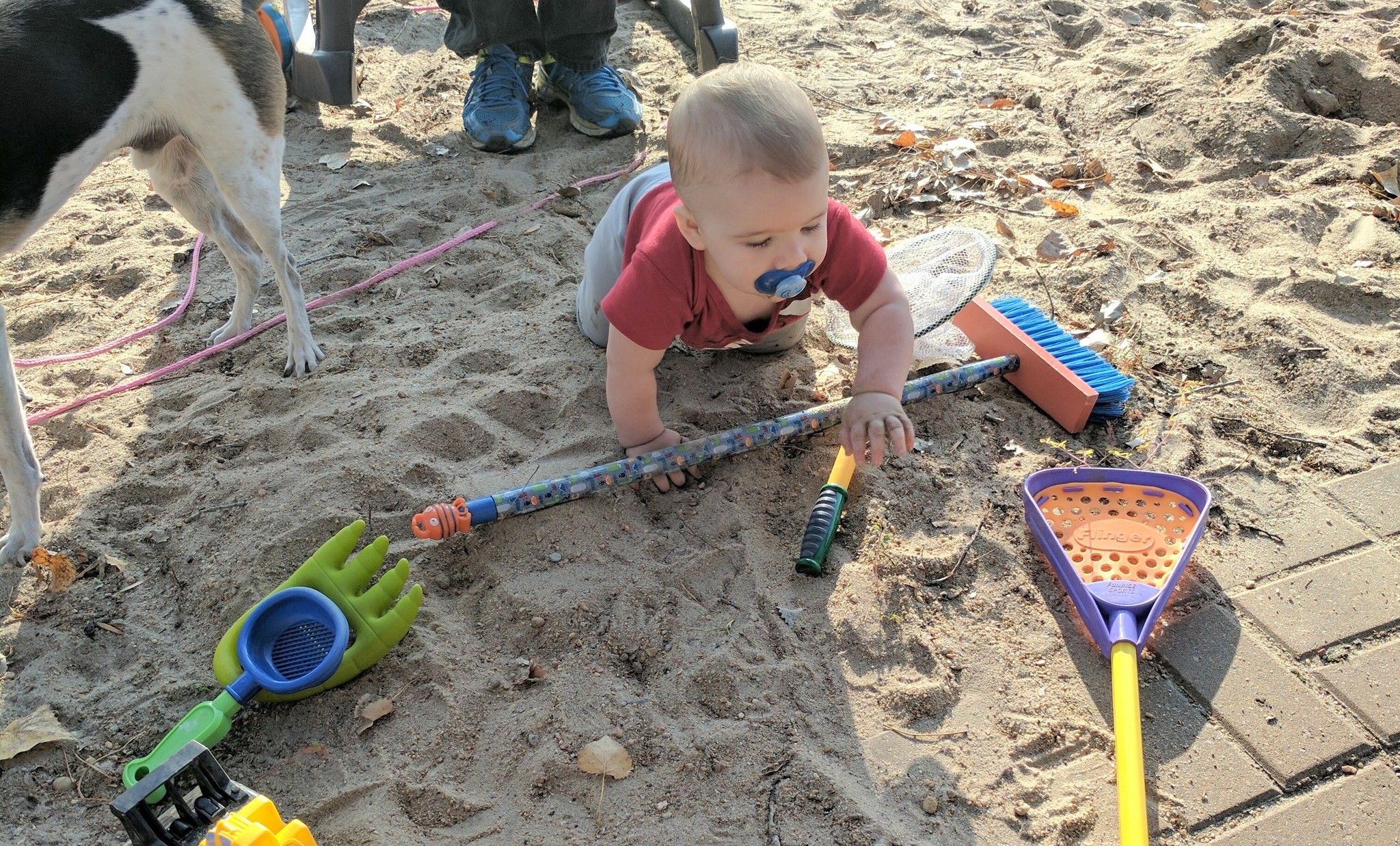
576;55;915;491
436;0;642;156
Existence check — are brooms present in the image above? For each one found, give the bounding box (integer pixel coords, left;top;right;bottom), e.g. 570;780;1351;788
408;288;1137;543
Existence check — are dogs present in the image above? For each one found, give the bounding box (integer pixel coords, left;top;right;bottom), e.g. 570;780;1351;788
1;0;327;574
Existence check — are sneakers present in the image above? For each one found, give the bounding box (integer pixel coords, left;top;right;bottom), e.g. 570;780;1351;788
535;50;641;138
461;41;538;153
